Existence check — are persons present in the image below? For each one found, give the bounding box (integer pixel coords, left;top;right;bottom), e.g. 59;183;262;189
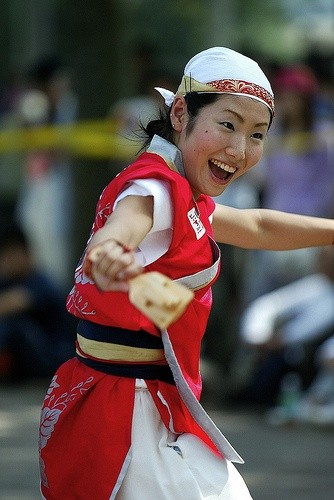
4;60;332;422
38;47;333;500
